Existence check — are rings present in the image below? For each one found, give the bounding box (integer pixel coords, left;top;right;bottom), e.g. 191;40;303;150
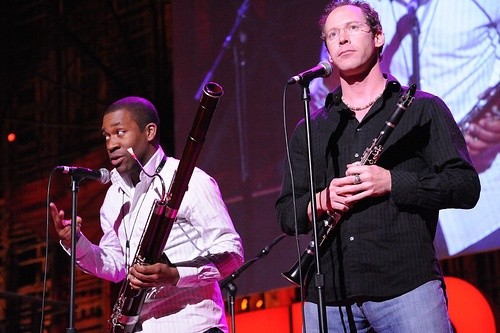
355;174;360;186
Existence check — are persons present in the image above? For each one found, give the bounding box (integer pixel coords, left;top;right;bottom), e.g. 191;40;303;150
50;97;244;333
276;0;481;333
308;1;500;258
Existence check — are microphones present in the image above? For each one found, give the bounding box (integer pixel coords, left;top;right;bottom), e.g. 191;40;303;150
287;61;333;85
56;165;112;185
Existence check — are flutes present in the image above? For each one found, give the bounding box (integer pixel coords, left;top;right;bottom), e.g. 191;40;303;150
279;82;417;289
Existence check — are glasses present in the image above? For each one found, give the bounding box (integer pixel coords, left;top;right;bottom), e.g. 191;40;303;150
324;21;368;40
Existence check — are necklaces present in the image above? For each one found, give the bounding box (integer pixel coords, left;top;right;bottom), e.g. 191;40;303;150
343;90;387;111
120;152;168;248
